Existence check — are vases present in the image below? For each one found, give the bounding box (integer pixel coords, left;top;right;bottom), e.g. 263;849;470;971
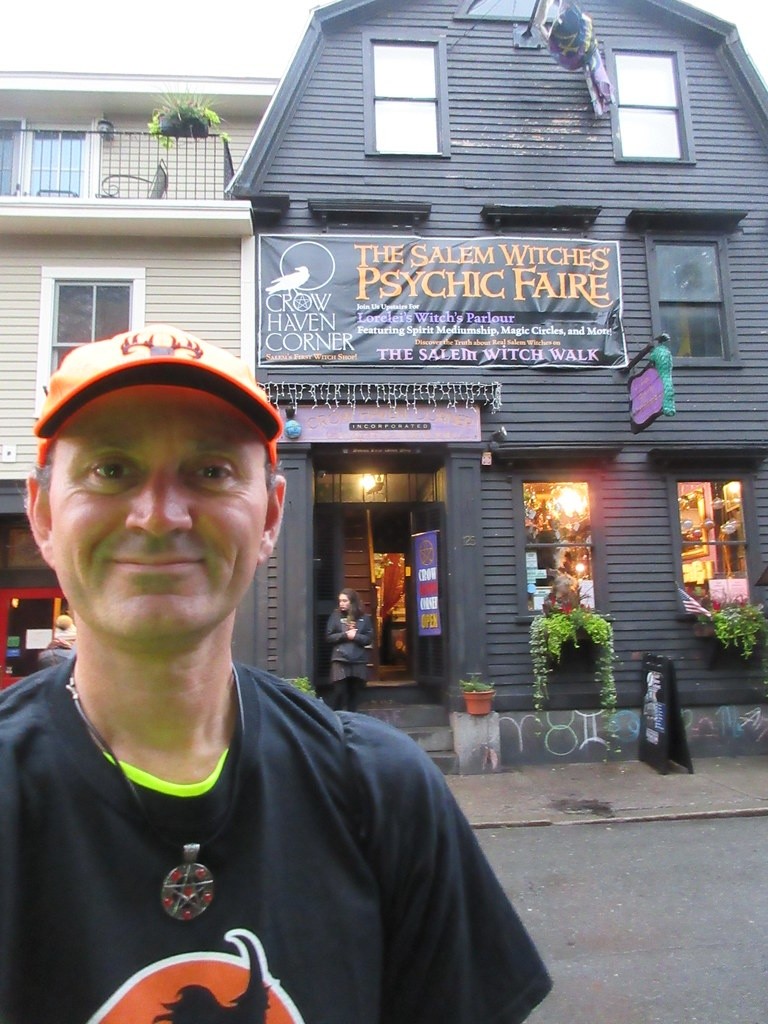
693;620;768;638
543;616;616;640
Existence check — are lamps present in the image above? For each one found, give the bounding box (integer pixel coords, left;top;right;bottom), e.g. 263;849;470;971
97;121;114;140
492;426;508;440
682;481;742;537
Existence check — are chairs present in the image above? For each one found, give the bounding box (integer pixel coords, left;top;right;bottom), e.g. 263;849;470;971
94;159;169;199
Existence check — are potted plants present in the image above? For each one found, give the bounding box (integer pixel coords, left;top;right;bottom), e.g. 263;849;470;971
458;675;496;714
146;80;233;149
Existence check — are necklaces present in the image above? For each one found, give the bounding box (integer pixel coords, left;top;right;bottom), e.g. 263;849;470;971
65;663;246;920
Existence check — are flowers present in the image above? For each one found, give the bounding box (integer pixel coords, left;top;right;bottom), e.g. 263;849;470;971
530;584;623;764
697;584;768;704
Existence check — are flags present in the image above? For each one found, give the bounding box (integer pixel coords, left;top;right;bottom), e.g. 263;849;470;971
678;588;712;616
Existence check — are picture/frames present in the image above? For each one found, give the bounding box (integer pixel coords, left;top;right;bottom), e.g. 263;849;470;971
678;486;710;561
721;481;740;513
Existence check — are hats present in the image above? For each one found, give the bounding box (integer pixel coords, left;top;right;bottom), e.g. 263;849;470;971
34;322;283;472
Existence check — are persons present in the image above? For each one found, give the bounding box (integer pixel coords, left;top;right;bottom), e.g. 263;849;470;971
0;321;554;1024
326;588;374;711
